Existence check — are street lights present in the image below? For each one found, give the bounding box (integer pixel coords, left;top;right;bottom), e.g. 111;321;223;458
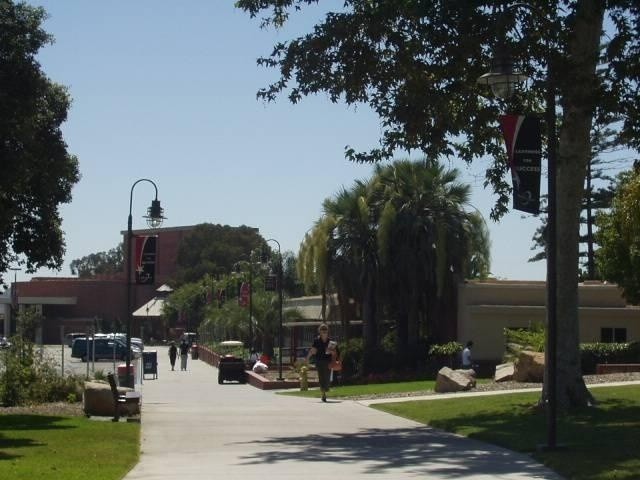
234;261;253;354
261;239;285;381
126;178;168;388
476;5;558;450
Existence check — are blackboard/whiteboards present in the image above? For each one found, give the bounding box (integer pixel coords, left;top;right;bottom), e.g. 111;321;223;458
142;351;157;374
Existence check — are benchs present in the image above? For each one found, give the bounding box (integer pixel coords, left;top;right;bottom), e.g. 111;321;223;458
107;372;140;422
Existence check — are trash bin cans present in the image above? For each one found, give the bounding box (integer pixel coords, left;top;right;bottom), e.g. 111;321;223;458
600;327;613;343
192;344;198;360
615;328;627;343
118;364;134;388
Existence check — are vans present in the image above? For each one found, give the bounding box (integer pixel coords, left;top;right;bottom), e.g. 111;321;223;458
66;332;144;361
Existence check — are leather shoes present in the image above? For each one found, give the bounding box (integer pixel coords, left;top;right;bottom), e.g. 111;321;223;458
321;394;327;403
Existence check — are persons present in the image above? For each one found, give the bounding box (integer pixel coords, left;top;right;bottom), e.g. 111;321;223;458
462;340;475;370
179;339;189;371
168;343;177;371
304;323;341;404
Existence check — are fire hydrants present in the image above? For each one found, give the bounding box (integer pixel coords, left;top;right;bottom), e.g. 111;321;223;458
299;365;309;392
